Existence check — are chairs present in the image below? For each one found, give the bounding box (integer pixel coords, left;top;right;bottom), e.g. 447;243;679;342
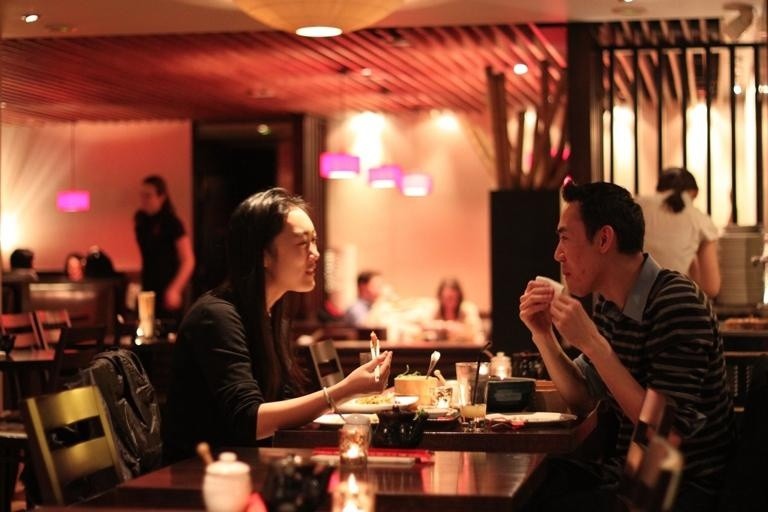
303;339;346;390
0;278;168;511
611;351;768;511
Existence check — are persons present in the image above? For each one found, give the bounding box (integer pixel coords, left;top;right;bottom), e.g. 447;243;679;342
339;268;396;338
162;186;394;455
632;163;723;302
414;279;487;349
63;248;89;279
515;178;737;505
3;245;39;314
131;172;197;320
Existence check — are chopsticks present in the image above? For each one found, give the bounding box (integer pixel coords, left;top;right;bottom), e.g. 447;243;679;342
369;341;381;383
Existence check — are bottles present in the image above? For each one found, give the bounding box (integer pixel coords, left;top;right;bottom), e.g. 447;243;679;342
490;352;512;378
202;453;252;512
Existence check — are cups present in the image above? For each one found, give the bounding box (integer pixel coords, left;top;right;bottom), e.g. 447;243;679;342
457;363;491;425
394;375;439;413
338;427;369;469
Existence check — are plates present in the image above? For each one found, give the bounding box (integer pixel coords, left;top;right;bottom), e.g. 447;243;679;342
485;409;578;425
314;415;379;426
340;397;421;411
421;406;456;420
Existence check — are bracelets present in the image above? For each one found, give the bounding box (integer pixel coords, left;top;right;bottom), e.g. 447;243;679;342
322;385;332;410
